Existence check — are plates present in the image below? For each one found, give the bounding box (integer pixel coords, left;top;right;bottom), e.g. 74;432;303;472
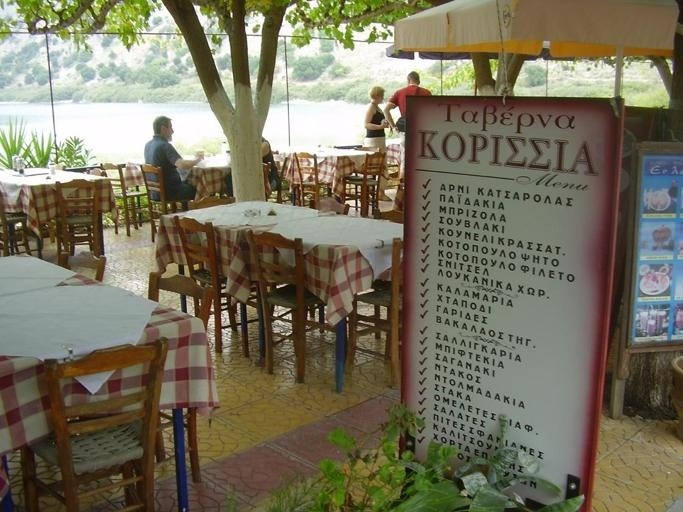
639;271;670;296
648;191;670;212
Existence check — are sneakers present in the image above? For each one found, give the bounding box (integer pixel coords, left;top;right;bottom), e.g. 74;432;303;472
378;194;392;200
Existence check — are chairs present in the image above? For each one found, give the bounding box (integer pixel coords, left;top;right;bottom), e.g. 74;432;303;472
148;271;214;482
21;337;167;512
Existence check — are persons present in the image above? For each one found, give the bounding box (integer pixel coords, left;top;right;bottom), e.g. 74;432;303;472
144;116;204;209
383;71;431;181
364;87;390;201
262;137;279;191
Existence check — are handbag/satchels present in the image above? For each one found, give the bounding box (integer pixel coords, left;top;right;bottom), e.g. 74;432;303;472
396;116;406;132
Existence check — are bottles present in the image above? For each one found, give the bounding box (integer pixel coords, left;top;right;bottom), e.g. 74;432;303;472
16;154;24;173
225;150;231;162
12;154;18;171
47;159;57;175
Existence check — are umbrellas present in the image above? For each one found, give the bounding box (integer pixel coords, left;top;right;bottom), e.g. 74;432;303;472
386;42;573;96
393;0;679;98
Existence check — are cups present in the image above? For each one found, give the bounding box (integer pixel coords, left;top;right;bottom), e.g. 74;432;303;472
639;311;667;337
676;305;683;329
